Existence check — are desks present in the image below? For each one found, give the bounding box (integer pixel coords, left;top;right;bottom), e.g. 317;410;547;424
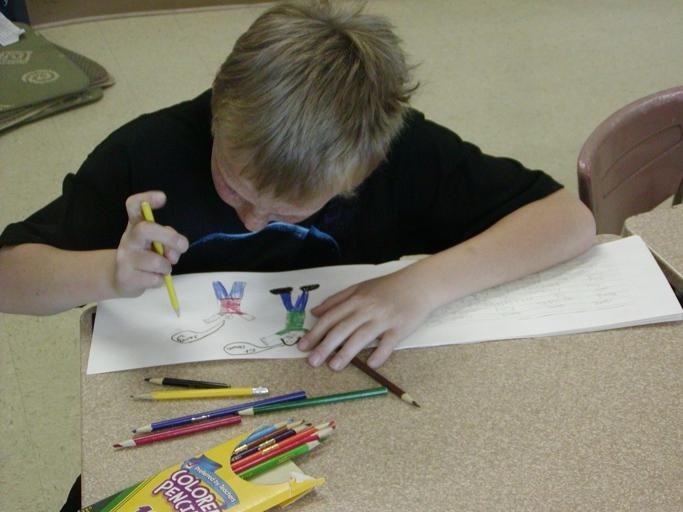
80;234;683;512
620;194;683;295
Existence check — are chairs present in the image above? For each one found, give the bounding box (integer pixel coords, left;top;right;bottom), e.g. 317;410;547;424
578;86;682;235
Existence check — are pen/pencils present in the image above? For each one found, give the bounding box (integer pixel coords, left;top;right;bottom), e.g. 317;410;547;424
113;377;388;480
320;335;421;408
141;201;180;318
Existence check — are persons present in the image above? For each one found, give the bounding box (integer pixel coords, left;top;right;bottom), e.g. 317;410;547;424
0;0;598;374
